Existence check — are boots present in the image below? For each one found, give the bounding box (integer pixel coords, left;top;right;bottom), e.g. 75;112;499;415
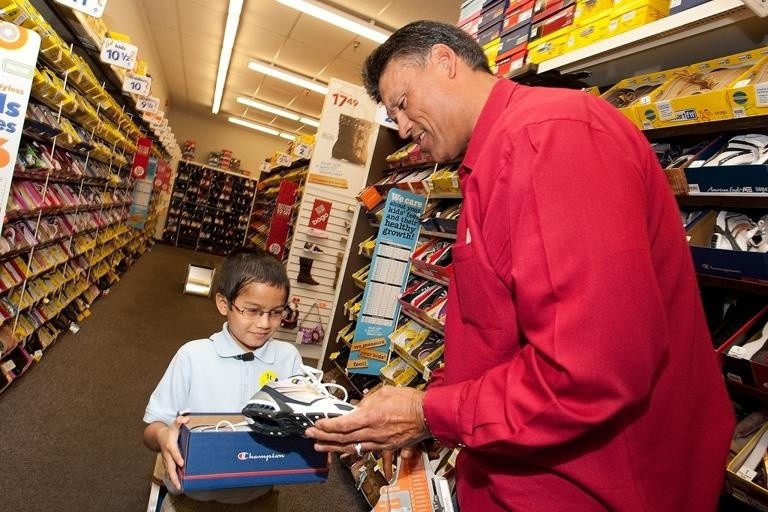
297;257;319;285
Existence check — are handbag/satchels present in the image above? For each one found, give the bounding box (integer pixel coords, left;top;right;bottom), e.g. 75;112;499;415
298;303;324;344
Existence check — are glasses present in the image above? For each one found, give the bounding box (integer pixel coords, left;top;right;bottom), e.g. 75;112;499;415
231;302;288;320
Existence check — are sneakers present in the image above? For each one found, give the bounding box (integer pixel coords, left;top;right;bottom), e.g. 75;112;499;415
396;321;444;370
648;133;768;168
606;65;755;109
304;242;323;254
241;372;360;438
701;288;768;364
190;420;252;432
386;359;426;387
307;228;328;238
726;410;768;489
418;241;454;265
441;204;460;220
402;280;447;319
680;209;768;253
373;167;434;184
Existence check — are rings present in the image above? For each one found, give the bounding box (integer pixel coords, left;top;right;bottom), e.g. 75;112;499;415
355;443;364;458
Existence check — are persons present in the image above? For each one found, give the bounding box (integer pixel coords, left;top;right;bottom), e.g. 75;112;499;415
304;19;737;511
142;244;332;512
